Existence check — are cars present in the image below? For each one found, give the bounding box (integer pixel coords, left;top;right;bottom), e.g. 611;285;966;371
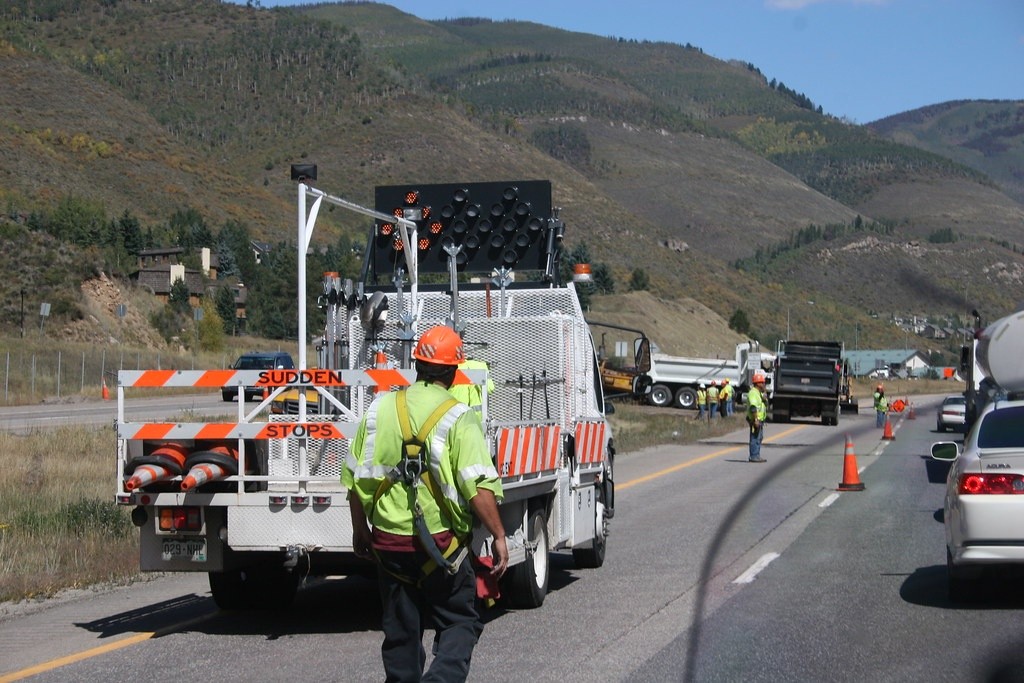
934;395;967;433
271;365;320;415
930;392;1024;598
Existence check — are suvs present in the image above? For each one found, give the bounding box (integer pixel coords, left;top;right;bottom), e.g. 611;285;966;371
221;351;298;402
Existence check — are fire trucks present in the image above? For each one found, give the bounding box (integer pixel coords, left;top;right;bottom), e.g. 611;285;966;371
113;162;656;616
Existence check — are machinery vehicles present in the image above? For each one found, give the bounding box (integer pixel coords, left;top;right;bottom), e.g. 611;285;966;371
594;329;860;426
958;307;1024;446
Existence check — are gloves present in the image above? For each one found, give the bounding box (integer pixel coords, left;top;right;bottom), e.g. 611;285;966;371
878;392;884;400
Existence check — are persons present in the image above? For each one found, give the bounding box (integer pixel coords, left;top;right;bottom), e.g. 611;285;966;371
340;326;509;683
873;385;889;428
745;373;767;463
694;378;735;421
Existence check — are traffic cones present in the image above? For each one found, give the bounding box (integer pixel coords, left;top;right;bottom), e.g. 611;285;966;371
180;439;264;493
125;440;193;492
906;402;916;419
903;395;910;406
372;348;391;399
102;378;111;400
881;411;897;441
262;386;269;400
837;434;865;491
888;395;894;412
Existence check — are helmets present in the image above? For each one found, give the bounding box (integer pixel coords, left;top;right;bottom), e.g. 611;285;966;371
700;383;705;388
711;380;716;385
752;374;765;383
877;385;882;392
725;378;730;382
721;380;726;385
413;326;465;365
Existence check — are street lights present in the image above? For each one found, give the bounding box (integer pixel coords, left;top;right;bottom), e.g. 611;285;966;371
786;300;815;340
194;283;244;358
905;320;927;380
855;315;878;380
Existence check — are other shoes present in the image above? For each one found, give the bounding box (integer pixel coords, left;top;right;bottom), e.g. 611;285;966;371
749;457;762;462
758;456;767;462
